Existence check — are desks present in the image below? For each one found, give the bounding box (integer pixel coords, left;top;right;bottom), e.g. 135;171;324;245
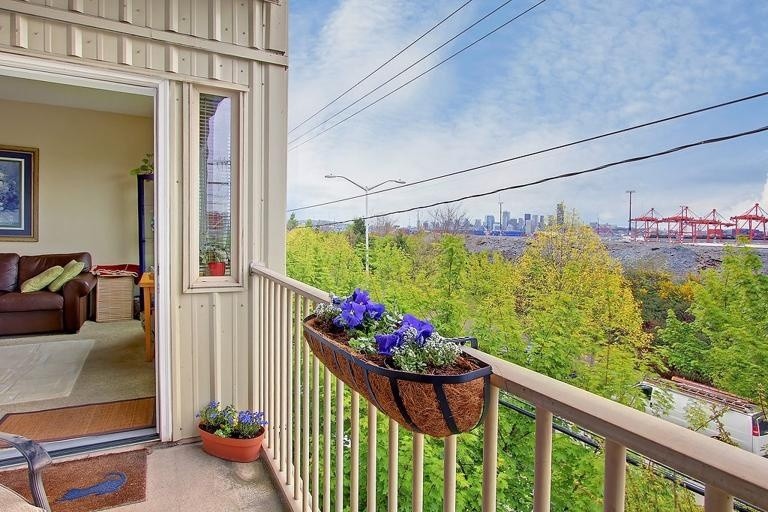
94;275;133;323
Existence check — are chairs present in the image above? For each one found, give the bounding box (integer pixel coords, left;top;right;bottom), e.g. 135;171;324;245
0;430;57;511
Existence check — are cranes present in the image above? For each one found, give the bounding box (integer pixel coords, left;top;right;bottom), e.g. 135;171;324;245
631;202;768;241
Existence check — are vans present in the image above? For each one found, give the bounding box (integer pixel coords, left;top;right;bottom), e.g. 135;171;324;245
611;378;768;459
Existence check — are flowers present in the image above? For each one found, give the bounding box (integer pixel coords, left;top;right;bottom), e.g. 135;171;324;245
197;401;270;438
313;289;461;371
199;245;232;262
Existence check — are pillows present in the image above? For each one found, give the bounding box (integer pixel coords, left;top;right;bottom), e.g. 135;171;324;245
21;259;85;293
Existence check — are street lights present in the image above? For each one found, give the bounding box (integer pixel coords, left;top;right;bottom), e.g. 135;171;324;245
498;202;503;230
325;174;406;273
625;190;635;231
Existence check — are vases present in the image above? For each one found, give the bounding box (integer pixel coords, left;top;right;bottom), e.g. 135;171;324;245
205;262;226;277
197;425;264;462
302;312;492;438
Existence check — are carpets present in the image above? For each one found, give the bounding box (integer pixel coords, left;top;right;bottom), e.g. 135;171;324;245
2;396;156;449
0;449;147;512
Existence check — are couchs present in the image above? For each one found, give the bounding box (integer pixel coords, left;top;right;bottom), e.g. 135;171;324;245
0;252;97;336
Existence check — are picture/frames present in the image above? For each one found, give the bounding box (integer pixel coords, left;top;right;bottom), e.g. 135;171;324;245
0;144;39;243
